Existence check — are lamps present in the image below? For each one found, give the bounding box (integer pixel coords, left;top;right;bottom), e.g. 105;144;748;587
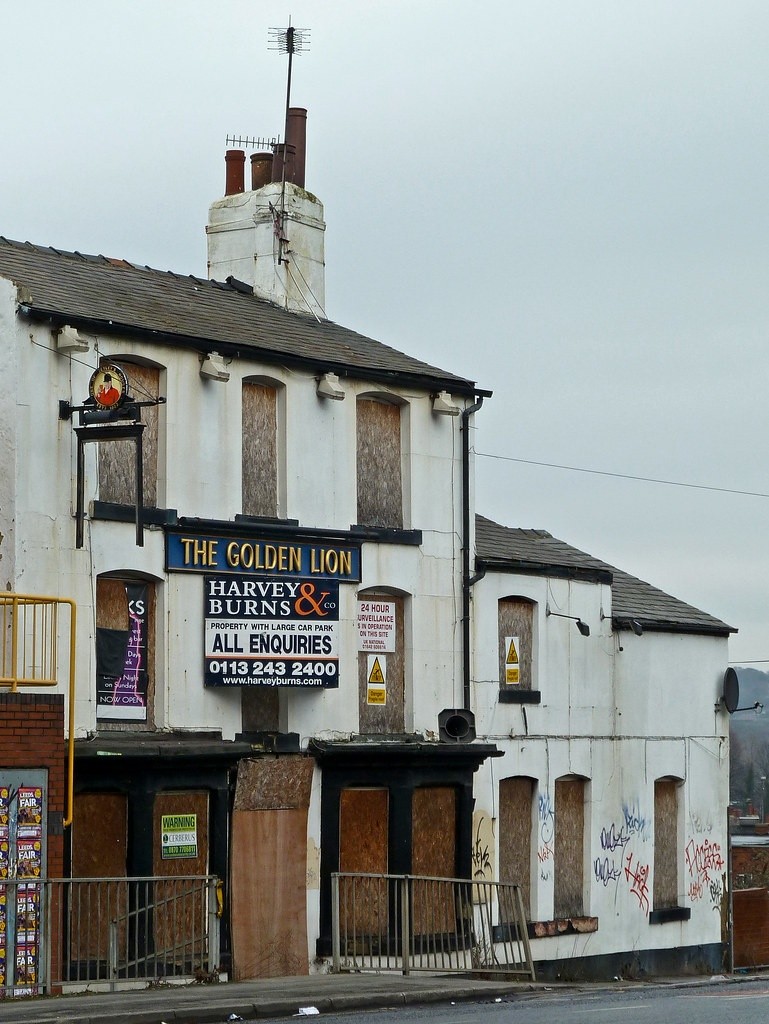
55;325;91;353
316;373;345;401
545;603;590;637
431;390;459;416
200;351;231;381
600;610;643;636
